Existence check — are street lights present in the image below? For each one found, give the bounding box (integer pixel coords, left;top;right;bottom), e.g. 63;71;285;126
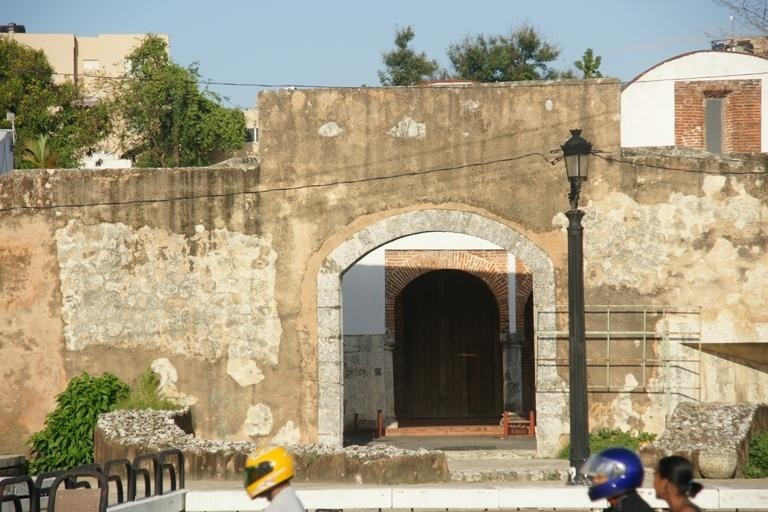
557;126;599;488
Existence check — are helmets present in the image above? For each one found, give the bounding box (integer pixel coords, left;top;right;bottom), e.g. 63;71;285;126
580;448;644;502
243;445;295;500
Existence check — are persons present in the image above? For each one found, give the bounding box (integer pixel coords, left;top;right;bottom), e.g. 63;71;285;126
240;441;308;511
579;446;654;511
654;454;705;511
